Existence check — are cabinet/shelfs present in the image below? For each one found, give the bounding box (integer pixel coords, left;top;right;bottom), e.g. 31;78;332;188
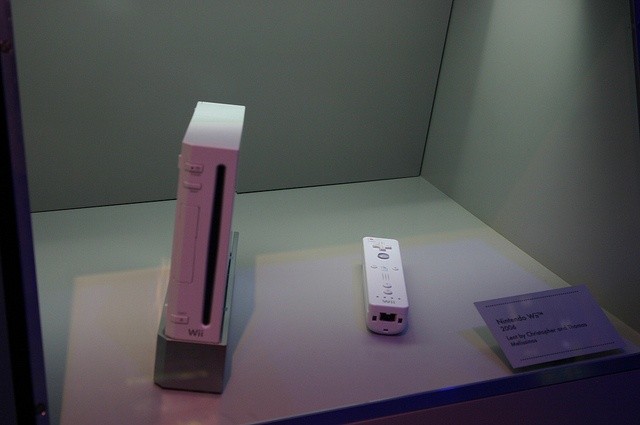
1;0;636;425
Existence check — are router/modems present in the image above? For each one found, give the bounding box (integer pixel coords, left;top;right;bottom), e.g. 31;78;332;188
165;98;247;343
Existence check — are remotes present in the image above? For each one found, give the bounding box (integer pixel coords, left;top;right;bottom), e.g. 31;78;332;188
361;234;412;337
